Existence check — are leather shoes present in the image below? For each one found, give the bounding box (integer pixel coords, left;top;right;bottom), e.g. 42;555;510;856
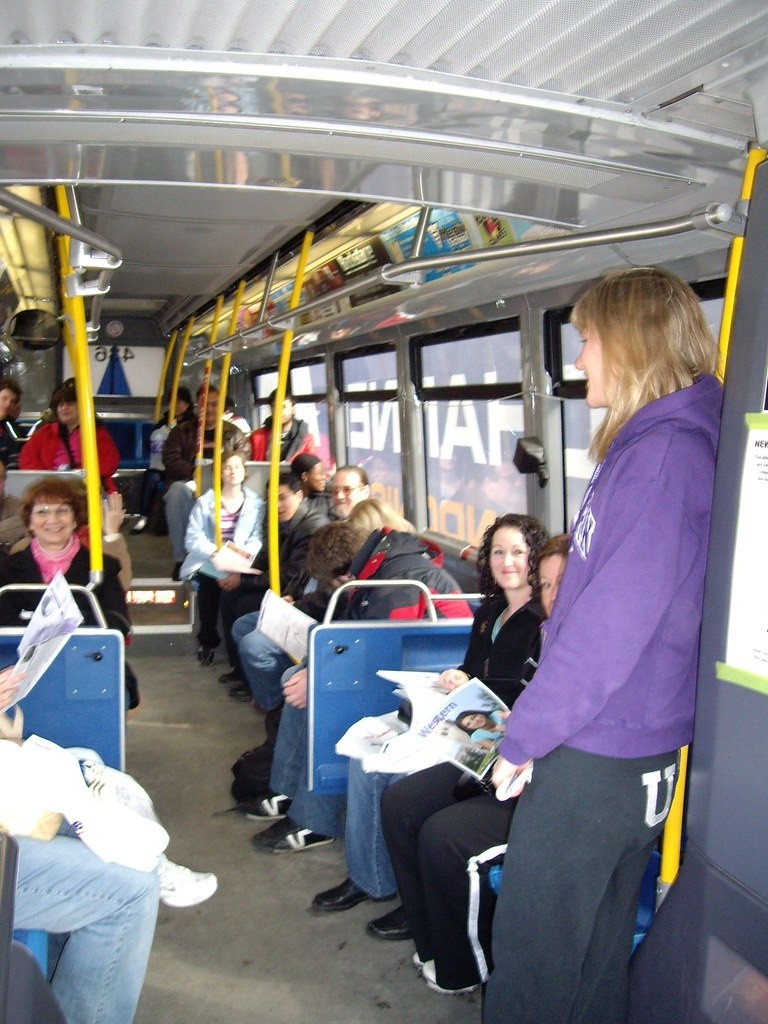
311;876;401;911
365;904;413;941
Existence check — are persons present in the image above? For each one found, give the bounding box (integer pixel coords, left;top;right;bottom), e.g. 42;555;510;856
0;378;29;465
129;388;195;534
455;710;508;748
477;269;731;1024
311;513;549;939
160;385;250;582
382;538;571;993
0;665;218;1024
20;386;119;495
1;459;142;708
218;455;473;852
252;388;314;462
179;452;266;667
224;398;251;435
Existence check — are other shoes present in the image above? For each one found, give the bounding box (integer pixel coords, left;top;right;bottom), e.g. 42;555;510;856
172;561;183;581
128;522;149;536
196;643;214;665
228;685;252;698
218;671;243;683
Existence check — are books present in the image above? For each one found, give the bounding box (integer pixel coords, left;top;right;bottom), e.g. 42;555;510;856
377;670;510;780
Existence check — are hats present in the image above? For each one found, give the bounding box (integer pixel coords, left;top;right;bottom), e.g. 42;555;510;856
289;453;320;474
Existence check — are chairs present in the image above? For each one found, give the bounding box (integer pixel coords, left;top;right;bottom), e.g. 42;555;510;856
0;414;669;1024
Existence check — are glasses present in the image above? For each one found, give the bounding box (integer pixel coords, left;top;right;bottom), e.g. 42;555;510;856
28;501;75;516
278;490;295;501
331;484;365;497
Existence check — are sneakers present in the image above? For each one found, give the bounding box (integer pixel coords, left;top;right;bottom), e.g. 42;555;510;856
159;859;219;909
236;788;294;821
252;813;336;854
